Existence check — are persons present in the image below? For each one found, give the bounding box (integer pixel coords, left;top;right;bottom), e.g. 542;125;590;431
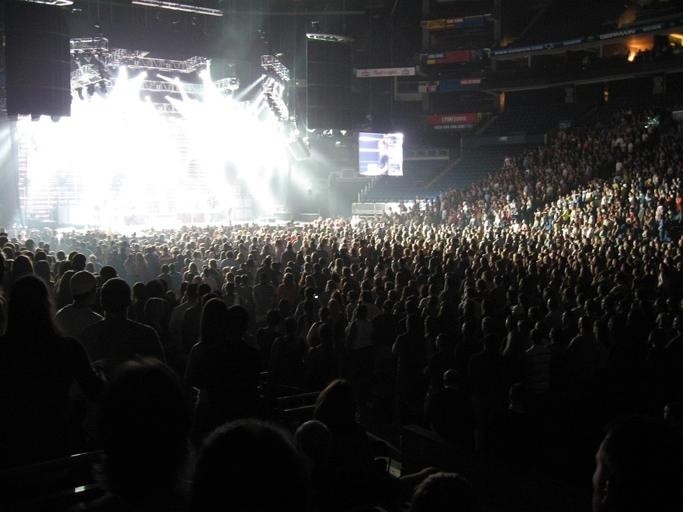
0;106;683;512
